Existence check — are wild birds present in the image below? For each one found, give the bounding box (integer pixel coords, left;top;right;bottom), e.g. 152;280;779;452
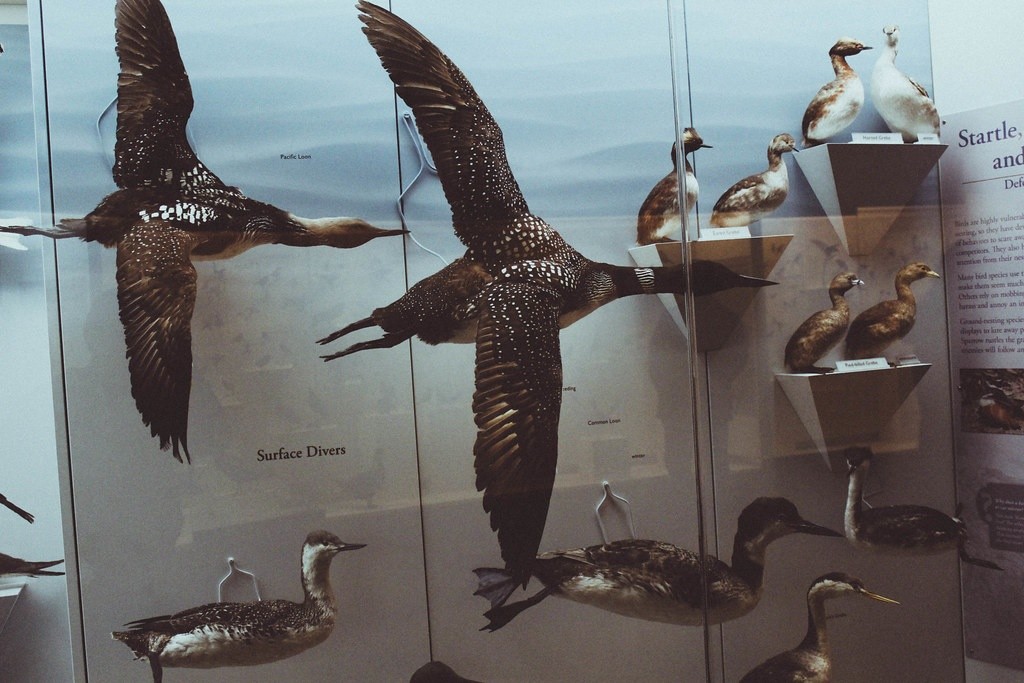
733;574;900;683
0;491;70;578
783;273;865;373
708;132;801;226
845;446;973;555
876;24;941;141
316;1;780;593
846;254;941;366
635;126;713;246
0;2;410;467
475;495;844;637
798;34;874;149
107;529;367;669
